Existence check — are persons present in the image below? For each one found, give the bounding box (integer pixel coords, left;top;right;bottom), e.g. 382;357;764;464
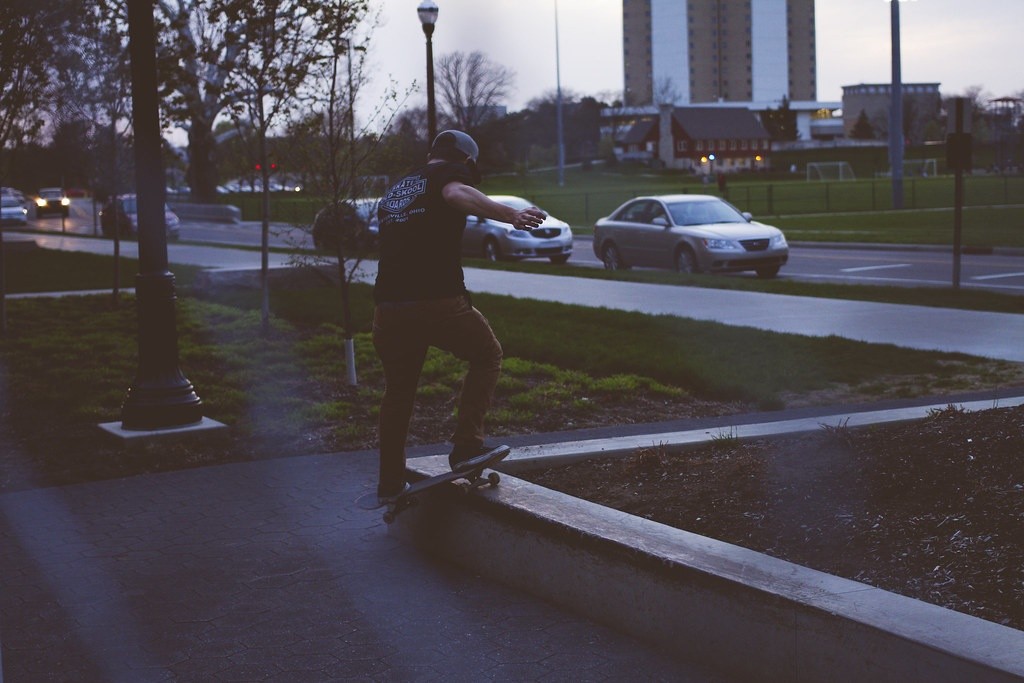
372;129;547;507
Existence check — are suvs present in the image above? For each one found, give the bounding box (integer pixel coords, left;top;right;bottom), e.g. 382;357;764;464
101;194;182;243
0;186;27;205
35;187;70;219
0;196;28;226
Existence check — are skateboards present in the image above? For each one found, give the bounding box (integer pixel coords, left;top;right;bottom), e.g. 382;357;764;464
358;446;513;524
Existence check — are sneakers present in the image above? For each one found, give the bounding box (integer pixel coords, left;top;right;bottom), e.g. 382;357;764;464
449;440;510;473
377;482;411;504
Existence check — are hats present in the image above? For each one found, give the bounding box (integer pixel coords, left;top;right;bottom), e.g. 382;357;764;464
432;130;478;165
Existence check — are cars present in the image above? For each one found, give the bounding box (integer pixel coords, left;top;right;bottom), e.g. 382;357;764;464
342;195;384;234
460;195;575;266
594;194;790;280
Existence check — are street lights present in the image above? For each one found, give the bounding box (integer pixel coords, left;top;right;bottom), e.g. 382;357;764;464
417;0;440;145
